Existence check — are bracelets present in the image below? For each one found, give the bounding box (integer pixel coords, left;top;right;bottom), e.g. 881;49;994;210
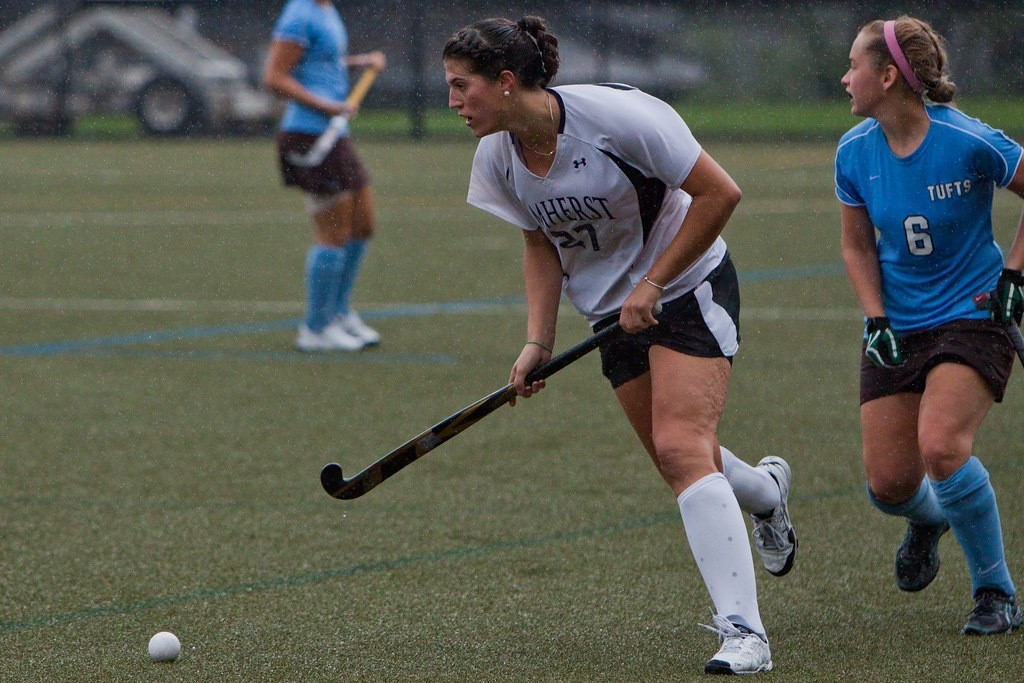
641;274;668;291
526;342;552;354
322;97;330;115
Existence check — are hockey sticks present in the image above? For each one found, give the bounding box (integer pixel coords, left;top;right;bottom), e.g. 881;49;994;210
321;304;666;500
287;67;377;170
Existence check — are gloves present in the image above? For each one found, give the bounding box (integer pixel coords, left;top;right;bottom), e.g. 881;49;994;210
864;316;908;371
988;268;1024;327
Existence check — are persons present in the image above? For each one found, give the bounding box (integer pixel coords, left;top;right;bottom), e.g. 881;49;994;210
263;1;386;352
834;17;1024;635
441;13;797;675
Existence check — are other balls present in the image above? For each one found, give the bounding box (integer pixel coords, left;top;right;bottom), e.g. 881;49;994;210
148;630;182;663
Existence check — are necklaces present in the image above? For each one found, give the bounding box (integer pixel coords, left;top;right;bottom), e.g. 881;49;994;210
519;90;557;156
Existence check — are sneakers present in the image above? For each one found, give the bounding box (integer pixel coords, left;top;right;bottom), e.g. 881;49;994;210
960;593;1024;637
748;455;800;576
895;517;951;593
704;631;774;676
292;306;381;354
